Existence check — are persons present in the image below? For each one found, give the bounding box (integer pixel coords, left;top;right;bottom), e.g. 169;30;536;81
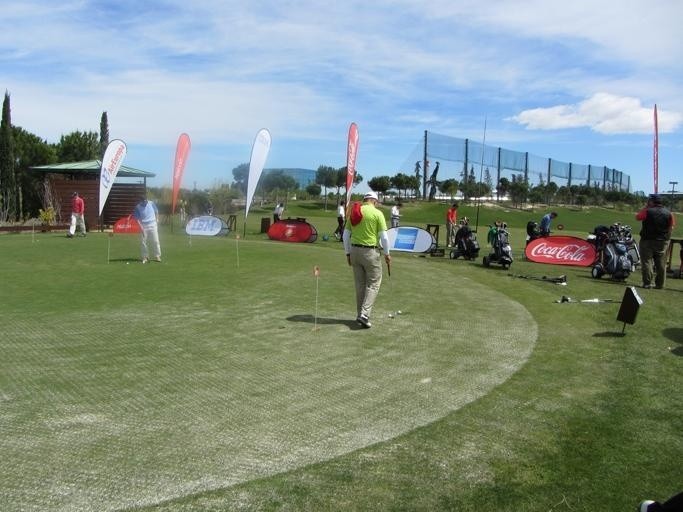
206;199;213;216
334;200;346;241
390;202;404;229
540;211;557;236
342;191;392;328
445;204;459;247
525;217;539;260
67;191;86;238
636;491;682;511
635;194;675;289
178;198;187;223
273;202;285;224
132;193;163;264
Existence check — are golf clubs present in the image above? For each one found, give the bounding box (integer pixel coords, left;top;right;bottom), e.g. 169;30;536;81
460;216;470;226
614;221;631;234
554;296;621;303
386;257;402;314
494;216;507;229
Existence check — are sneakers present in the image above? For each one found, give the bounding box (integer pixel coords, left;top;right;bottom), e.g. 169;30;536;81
357;313;372;328
155;256;162;262
643;282;650;288
653;284;664;289
142;257;148;263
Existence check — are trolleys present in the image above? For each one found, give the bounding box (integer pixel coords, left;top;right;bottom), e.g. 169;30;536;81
447;225;481;262
482;223;514;269
587;226;634;280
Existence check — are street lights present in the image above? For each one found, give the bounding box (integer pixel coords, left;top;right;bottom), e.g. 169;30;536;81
667;181;680;210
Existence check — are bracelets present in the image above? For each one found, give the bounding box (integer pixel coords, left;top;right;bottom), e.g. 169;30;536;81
345;254;349;256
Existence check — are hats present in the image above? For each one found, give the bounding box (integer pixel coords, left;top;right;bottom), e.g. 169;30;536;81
71;192;79;197
140;194;148;204
363;192;378;200
649;194;660;200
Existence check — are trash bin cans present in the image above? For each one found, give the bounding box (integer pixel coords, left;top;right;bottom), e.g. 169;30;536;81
261;218;270;233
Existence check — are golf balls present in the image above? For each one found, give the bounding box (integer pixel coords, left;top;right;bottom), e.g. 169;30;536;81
126;261;130;263
389;314;393;318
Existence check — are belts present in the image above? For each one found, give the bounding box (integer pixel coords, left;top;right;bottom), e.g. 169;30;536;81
351;244;379;249
